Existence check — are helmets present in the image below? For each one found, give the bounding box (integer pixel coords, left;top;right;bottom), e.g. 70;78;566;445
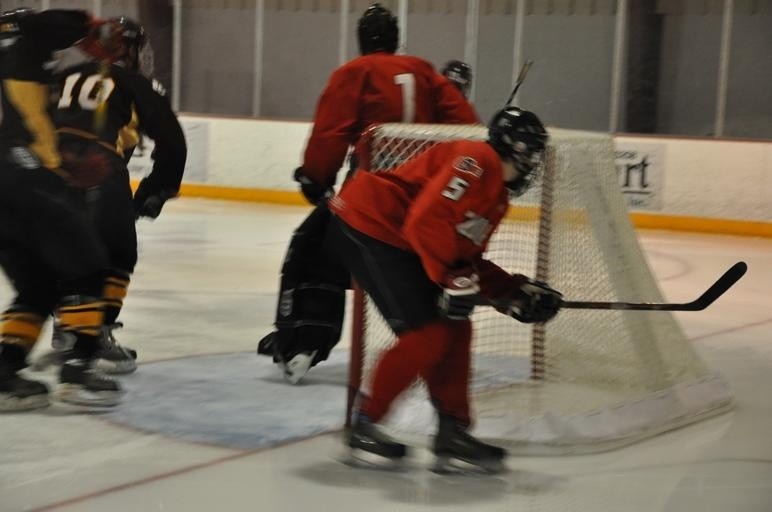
444;61;472;98
358;6;397;54
112;15;147;72
489;105;548;192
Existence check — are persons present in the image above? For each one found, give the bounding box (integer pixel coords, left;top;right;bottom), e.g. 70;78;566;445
441;59;473;97
256;2;484;367
0;6;186;406
325;105;561;460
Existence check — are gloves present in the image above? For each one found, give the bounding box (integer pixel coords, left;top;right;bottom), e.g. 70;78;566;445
499;274;565;324
134;179;168;221
292;168;335;204
435;261;480;319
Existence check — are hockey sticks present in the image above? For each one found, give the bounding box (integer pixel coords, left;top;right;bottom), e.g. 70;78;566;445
562;261;747;312
503;59;531;107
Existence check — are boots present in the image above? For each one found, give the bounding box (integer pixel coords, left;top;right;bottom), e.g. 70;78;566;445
346;409;407;458
0;314;137;398
434;410;506;459
